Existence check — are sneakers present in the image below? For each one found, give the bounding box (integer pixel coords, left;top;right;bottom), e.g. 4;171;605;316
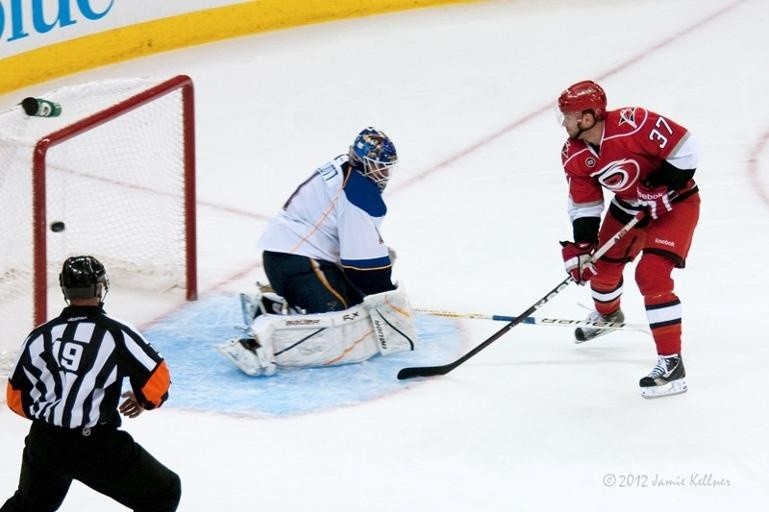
576;307;624;341
640;353;685;386
222;336;263;373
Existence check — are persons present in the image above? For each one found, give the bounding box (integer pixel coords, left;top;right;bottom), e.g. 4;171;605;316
0;252;182;511
556;79;712;391
217;125;416;376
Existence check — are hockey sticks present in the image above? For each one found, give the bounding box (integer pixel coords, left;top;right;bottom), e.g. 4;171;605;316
398;209;644;380
415;307;654;341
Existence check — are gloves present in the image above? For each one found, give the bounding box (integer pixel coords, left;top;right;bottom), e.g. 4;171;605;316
559;240;599;286
637;183;673;221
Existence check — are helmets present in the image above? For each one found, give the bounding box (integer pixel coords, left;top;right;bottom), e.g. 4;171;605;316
350;128;397;193
558;79;607;123
59;256;110;300
